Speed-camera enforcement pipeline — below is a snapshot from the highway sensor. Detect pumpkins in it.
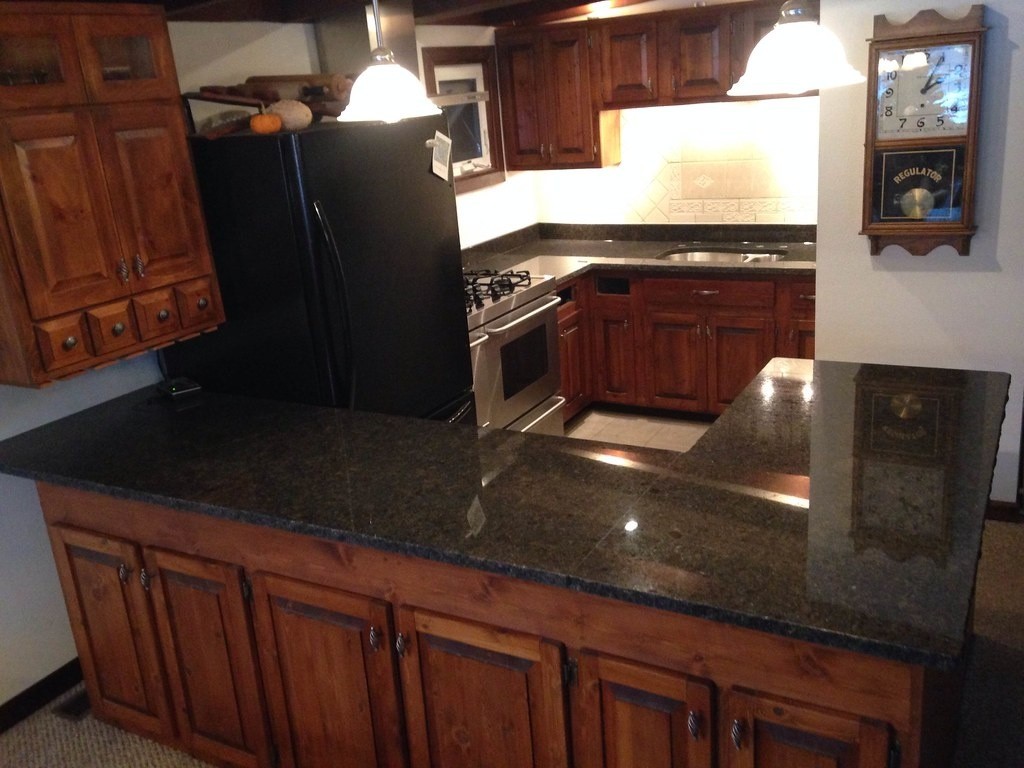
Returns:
[250,101,282,135]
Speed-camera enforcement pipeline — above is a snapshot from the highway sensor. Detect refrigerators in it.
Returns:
[173,113,477,426]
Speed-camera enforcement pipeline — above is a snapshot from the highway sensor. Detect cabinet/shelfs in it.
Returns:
[36,482,934,768]
[494,21,623,170]
[0,0,229,390]
[586,271,816,416]
[555,274,592,424]
[588,0,820,110]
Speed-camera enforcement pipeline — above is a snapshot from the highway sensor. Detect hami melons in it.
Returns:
[262,99,312,131]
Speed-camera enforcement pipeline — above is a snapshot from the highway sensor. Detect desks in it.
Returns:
[0,356,1012,768]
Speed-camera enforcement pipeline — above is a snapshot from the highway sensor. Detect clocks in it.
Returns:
[856,4,994,259]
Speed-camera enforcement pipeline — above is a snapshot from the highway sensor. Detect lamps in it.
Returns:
[335,0,443,124]
[726,0,867,96]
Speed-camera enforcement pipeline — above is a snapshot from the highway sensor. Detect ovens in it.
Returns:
[468,290,565,437]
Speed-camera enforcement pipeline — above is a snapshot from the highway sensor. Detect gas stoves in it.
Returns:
[462,266,557,332]
